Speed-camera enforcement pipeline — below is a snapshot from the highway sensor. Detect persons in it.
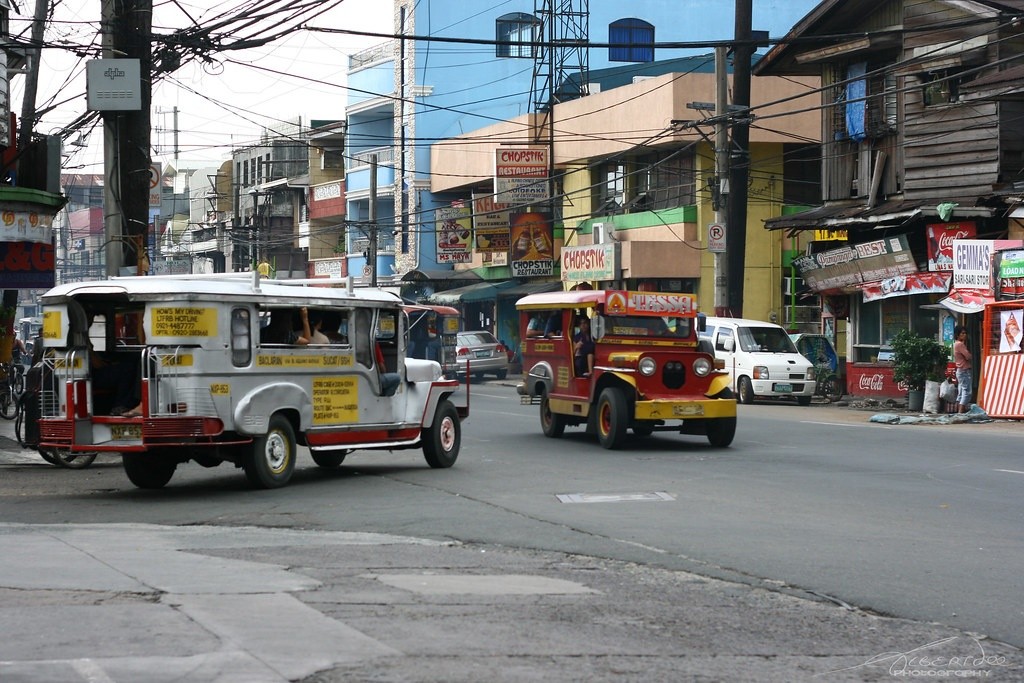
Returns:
[259,306,402,397]
[121,401,143,417]
[11,333,29,382]
[30,328,52,367]
[524,307,668,377]
[953,326,973,415]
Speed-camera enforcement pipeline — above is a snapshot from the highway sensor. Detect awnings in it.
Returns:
[431,279,517,305]
[761,201,949,229]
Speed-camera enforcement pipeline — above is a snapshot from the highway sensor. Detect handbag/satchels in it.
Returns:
[939,375,957,403]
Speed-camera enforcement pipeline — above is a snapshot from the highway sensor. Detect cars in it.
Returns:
[456,330,510,383]
[18,317,42,364]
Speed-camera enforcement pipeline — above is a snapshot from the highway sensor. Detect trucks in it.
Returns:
[33,263,471,491]
[515,289,741,451]
[382,302,460,378]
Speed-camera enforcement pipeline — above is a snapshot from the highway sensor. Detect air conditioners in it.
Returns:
[580,82,601,98]
[592,223,615,245]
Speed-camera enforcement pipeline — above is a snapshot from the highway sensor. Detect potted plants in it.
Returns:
[332,242,345,257]
[888,329,952,409]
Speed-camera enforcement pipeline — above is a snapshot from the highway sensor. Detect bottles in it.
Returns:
[451,221,469,239]
[439,226,447,248]
[516,222,530,257]
[447,224,459,243]
[928,227,940,263]
[532,222,547,252]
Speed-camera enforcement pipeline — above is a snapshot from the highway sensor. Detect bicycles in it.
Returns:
[0,352,28,421]
[801,350,845,402]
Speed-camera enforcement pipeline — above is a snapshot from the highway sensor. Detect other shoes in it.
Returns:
[583,373,591,378]
[122,409,143,417]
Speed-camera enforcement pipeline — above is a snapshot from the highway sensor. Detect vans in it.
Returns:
[692,316,817,407]
